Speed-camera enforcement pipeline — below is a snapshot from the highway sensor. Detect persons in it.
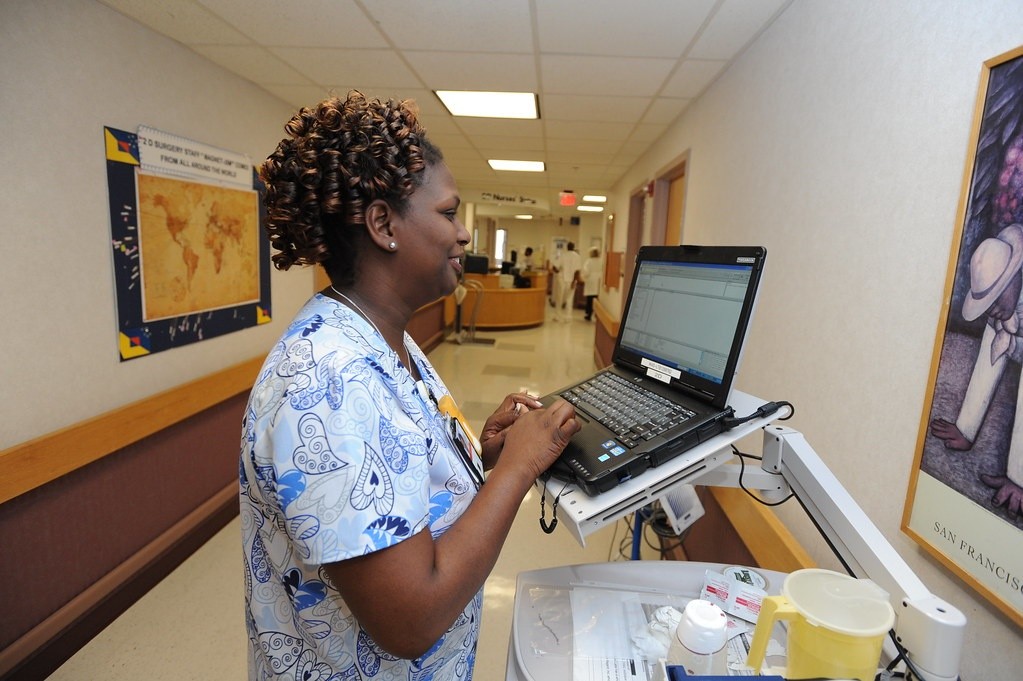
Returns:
[240,88,582,681]
[582,248,603,322]
[458,433,469,456]
[516,247,533,288]
[552,242,582,323]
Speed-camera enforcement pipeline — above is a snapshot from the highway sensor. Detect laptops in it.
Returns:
[521,244,768,498]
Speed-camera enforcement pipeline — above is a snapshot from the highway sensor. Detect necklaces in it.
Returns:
[329,282,412,376]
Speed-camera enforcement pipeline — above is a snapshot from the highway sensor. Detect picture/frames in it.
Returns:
[901,45,1023,627]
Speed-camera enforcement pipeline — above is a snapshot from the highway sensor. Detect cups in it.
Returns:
[666,599,727,675]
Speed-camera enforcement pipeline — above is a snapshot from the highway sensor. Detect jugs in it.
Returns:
[746,568,895,681]
